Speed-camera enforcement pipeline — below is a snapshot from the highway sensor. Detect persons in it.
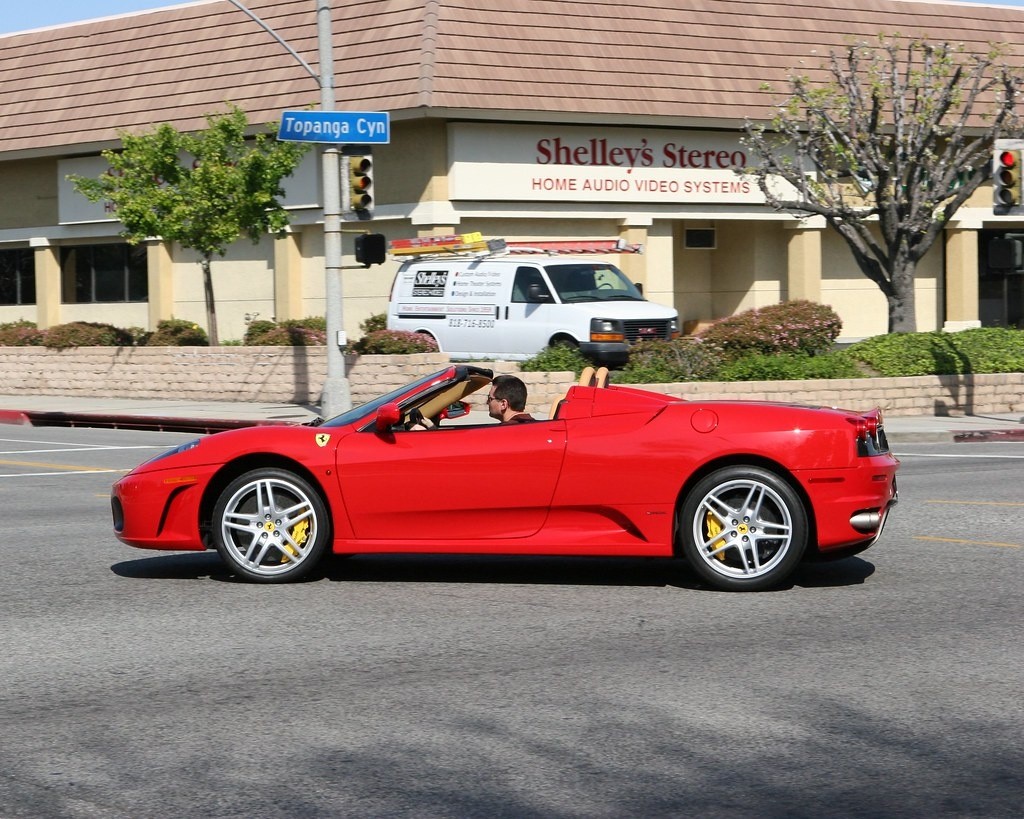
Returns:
[485,374,534,426]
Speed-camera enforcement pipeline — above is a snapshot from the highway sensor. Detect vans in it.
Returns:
[386,249,680,363]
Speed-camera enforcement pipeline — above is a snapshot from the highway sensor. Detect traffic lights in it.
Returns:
[338,150,375,223]
[355,233,385,264]
[993,136,1024,215]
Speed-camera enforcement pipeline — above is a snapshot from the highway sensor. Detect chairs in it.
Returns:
[550,366,610,419]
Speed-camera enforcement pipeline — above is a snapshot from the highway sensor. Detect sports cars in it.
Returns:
[112,363,904,592]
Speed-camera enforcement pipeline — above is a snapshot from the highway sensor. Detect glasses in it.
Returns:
[488,395,510,406]
